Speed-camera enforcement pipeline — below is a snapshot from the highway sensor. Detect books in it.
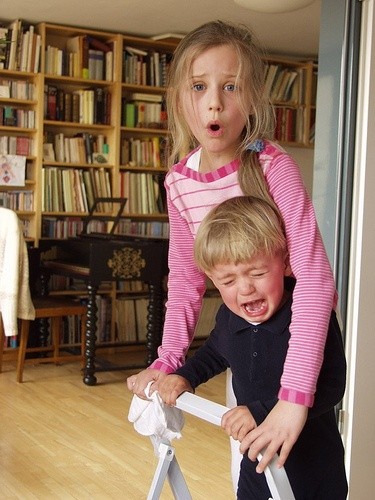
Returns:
[1,165,170,349]
[0,20,318,172]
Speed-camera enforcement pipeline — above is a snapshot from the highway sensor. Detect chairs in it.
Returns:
[0,206,89,384]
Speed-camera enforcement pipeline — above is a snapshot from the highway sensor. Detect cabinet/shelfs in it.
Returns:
[0,21,319,356]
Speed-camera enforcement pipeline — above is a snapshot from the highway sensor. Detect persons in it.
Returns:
[158,196,348,500]
[127,18,342,500]
